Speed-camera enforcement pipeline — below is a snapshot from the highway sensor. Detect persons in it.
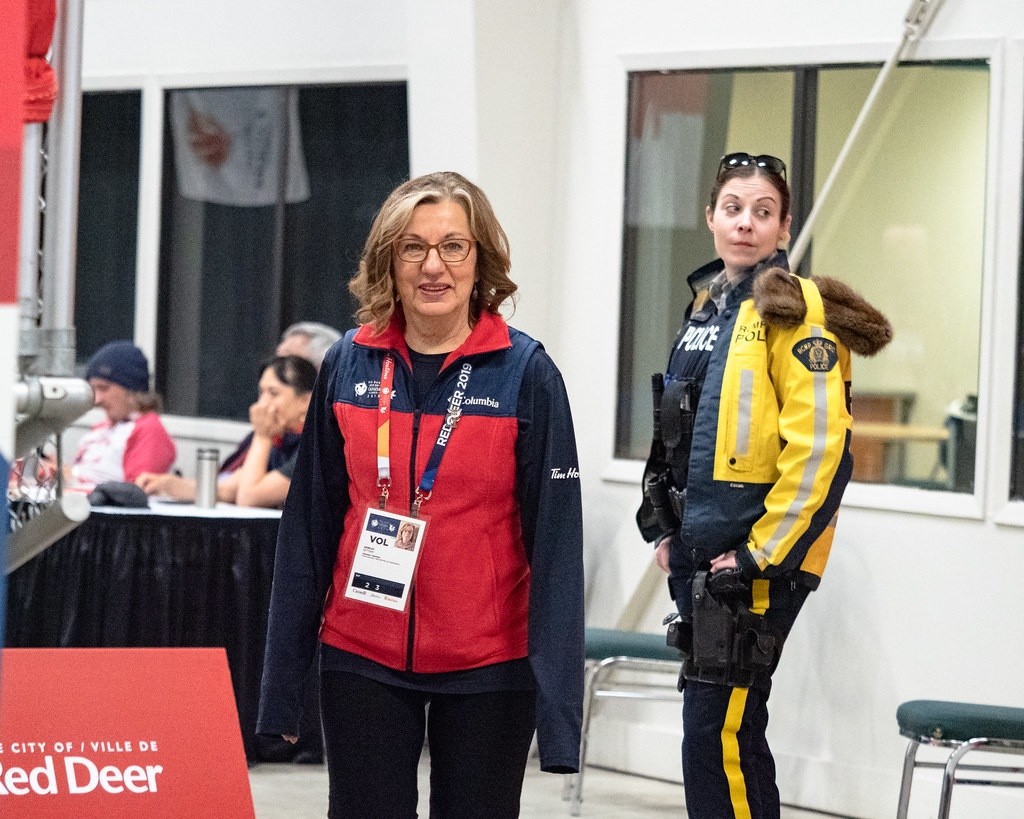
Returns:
[65,341,174,491]
[637,154,856,819]
[134,322,344,507]
[396,523,416,551]
[257,171,586,819]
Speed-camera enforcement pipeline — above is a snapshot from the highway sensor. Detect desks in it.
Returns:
[6,493,322,772]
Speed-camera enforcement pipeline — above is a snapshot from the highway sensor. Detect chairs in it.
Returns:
[895,700,1024,819]
[563,629,681,818]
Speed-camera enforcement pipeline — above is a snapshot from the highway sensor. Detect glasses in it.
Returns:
[716,152,787,184]
[392,238,478,263]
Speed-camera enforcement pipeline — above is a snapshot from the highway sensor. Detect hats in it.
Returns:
[753,268,893,359]
[85,341,150,391]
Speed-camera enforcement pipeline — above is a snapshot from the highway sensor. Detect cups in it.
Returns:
[197,447,219,507]
